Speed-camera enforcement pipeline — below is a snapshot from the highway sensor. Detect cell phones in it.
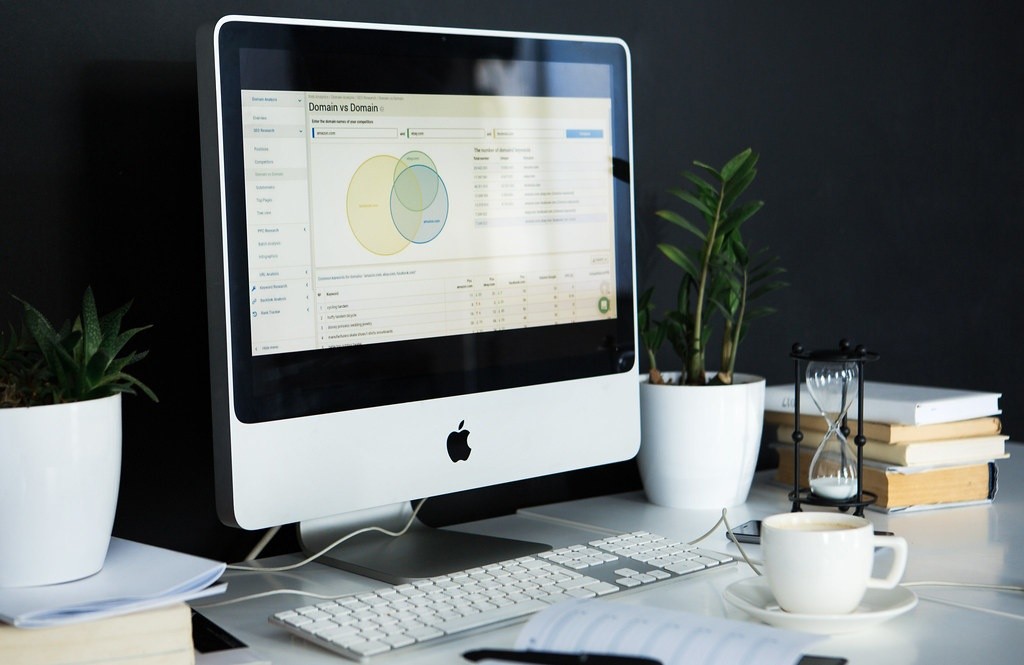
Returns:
[726,520,894,552]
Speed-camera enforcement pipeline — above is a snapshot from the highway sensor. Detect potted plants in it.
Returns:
[0,285,159,590]
[636,146,799,510]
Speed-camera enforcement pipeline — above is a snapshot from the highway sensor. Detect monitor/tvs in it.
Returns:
[195,13,642,588]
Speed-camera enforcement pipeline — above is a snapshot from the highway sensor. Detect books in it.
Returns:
[764,380,1010,513]
[0,580,230,663]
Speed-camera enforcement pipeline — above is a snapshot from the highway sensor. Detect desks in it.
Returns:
[193,437,1024,665]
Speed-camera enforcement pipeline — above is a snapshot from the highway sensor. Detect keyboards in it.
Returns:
[267,531,740,665]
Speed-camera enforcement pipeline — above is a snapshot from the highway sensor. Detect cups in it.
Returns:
[760,511,908,615]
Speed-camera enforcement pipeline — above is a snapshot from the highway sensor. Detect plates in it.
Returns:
[722,575,919,635]
